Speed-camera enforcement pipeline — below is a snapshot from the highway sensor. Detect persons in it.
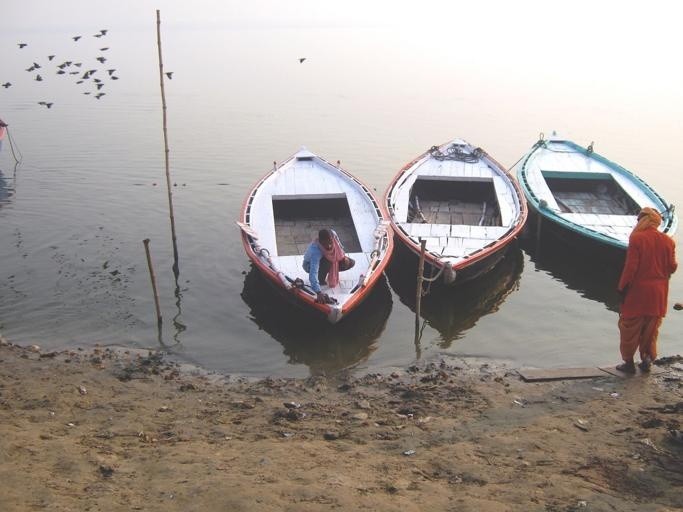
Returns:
[616,208,677,375]
[301,229,355,303]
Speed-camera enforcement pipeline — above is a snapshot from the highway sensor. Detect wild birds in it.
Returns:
[164,71,173,79]
[300,56,306,63]
[24,29,120,108]
[1,81,12,90]
[18,42,27,49]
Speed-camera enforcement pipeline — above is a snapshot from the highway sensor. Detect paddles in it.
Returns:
[236,220,293,290]
[361,221,391,287]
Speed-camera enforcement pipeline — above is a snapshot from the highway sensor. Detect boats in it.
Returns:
[382,136,528,284]
[239,149,395,325]
[518,138,679,275]
